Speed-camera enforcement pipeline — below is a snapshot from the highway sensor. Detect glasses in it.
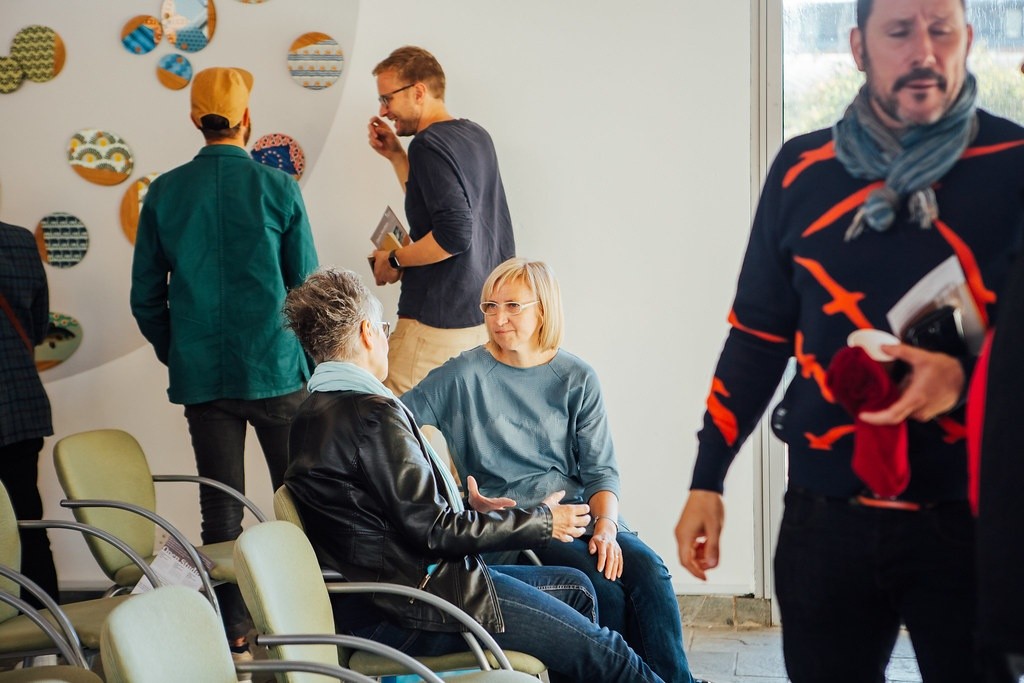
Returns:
[378,84,415,105]
[371,321,390,340]
[479,301,540,315]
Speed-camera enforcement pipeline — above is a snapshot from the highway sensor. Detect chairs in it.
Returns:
[0,422,552,683]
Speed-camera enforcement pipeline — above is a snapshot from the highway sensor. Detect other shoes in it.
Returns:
[230,640,254,662]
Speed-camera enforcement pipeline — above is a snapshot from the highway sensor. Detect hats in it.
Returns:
[191,66,254,131]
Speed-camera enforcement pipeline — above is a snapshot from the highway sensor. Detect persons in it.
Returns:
[283,257,705,683]
[673,0,1024,683]
[0,221,60,671]
[367,46,516,398]
[129,67,320,674]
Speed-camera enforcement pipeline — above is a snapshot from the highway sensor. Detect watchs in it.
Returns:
[388,249,402,270]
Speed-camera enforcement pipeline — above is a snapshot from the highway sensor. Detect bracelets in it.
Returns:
[593,515,619,531]
